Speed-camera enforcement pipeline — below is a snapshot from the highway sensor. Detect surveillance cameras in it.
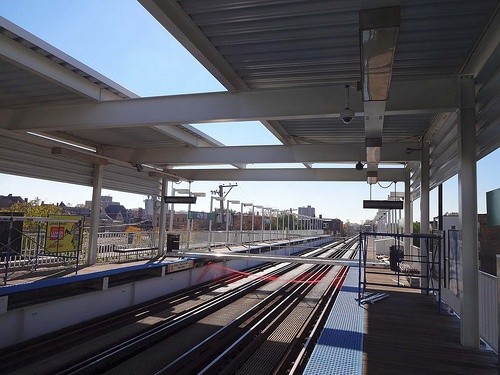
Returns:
[341,86,355,124]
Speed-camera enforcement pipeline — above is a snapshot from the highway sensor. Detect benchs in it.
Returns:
[395,261,421,288]
[113,246,158,263]
